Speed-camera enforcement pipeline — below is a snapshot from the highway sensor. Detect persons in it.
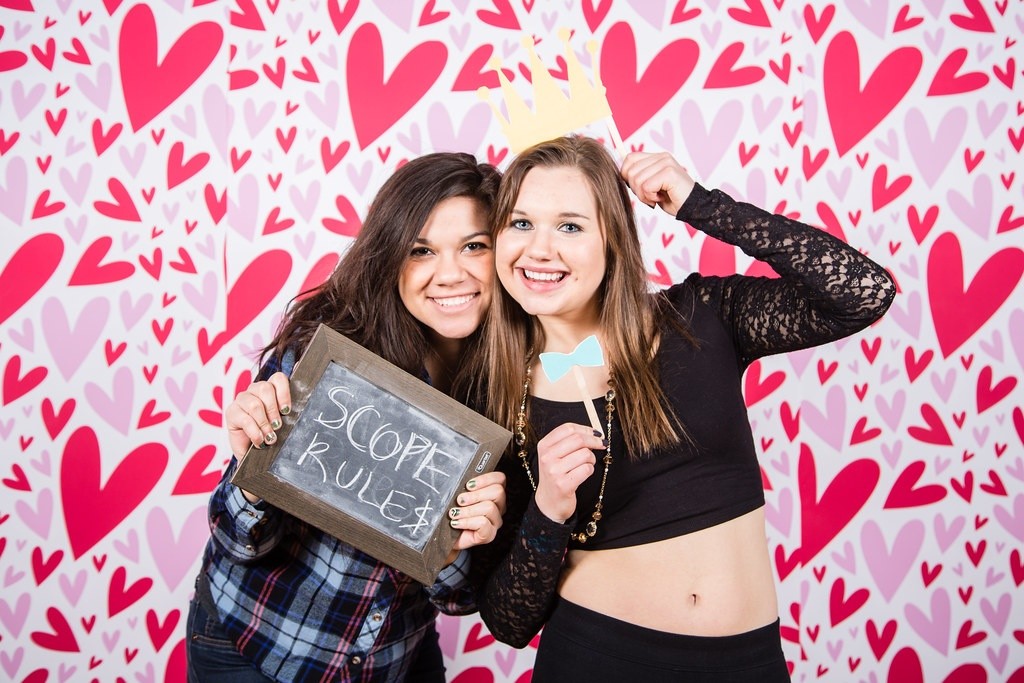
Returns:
[184,150,534,683]
[476,133,897,682]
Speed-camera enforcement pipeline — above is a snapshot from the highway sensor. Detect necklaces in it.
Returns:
[517,343,619,543]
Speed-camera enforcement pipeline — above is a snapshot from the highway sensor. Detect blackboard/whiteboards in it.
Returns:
[226,322,515,592]
